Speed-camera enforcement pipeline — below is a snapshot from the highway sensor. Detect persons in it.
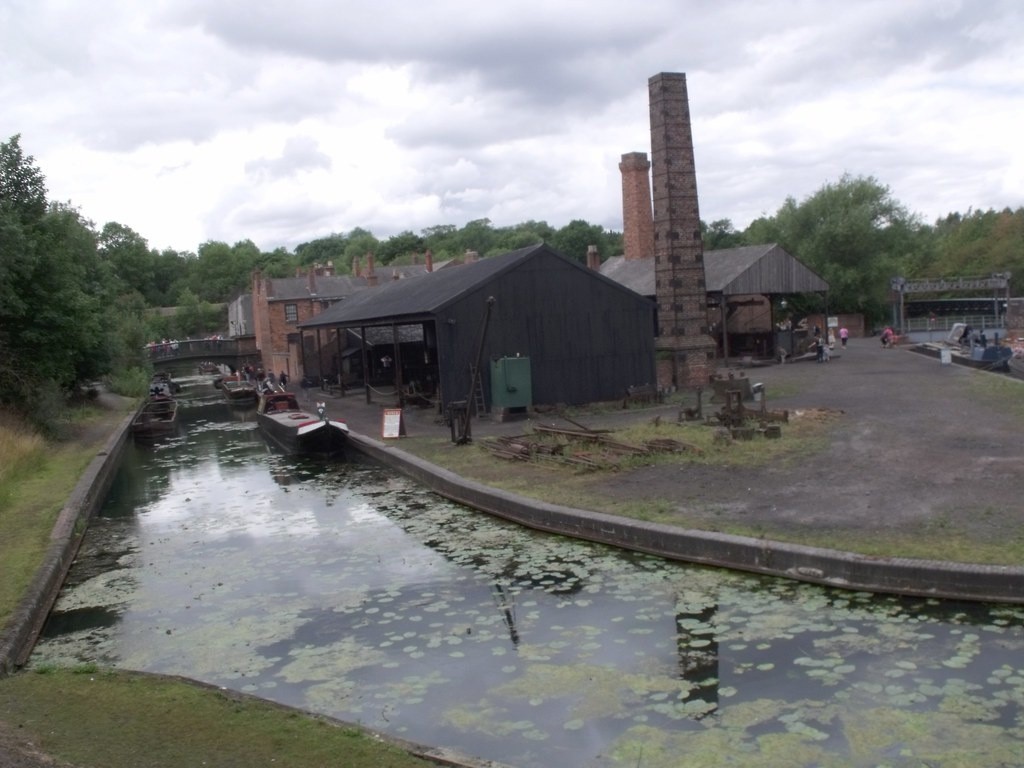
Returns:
[187,335,195,352]
[828,328,836,348]
[815,333,824,363]
[381,356,392,384]
[145,338,182,358]
[879,325,897,348]
[929,311,936,330]
[967,329,987,347]
[204,334,223,350]
[839,325,848,349]
[234,364,290,390]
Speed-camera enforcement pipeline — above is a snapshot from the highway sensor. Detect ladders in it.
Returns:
[469,363,486,417]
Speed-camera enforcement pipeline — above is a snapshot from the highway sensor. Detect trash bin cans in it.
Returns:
[447,400,470,443]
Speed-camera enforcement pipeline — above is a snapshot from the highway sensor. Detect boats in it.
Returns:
[150,370,175,401]
[199,361,222,376]
[132,397,178,435]
[915,322,1014,372]
[222,379,256,400]
[256,391,349,462]
